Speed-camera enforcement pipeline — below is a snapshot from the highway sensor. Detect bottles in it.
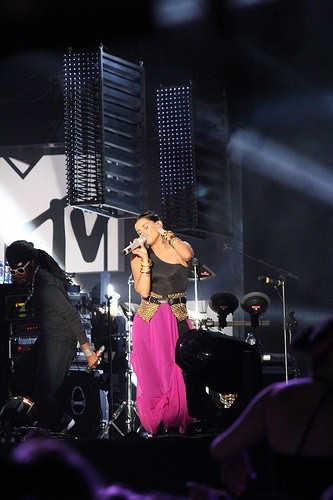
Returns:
[0,261,4,283]
[5,261,12,285]
[100,417,108,437]
[245,333,256,345]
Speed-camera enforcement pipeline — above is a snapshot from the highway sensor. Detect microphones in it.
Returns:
[85,345,105,373]
[122,237,146,255]
[257,276,282,286]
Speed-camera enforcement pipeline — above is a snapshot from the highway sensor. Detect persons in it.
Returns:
[129,211,195,436]
[209,312,333,500]
[4,241,103,432]
[0,437,168,500]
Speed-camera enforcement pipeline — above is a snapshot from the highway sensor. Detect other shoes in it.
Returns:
[55,414,75,438]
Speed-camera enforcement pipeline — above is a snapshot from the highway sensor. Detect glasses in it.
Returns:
[8,261,30,276]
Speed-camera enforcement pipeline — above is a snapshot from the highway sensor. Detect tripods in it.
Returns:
[96,273,141,439]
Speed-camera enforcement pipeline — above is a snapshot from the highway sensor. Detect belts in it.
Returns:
[141,295,187,305]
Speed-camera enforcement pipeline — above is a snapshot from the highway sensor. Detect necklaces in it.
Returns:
[26,265,41,298]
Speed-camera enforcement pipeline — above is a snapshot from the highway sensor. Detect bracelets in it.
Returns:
[83,348,93,357]
[161,230,179,246]
[140,259,152,276]
